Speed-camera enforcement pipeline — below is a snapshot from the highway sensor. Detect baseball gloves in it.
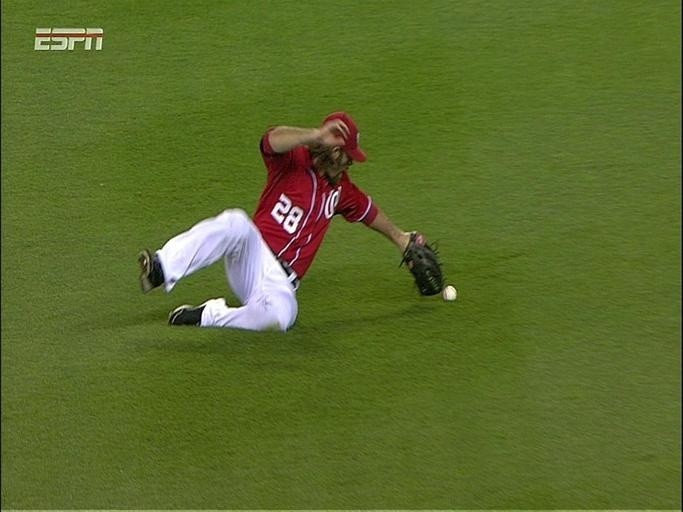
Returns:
[398,231,447,295]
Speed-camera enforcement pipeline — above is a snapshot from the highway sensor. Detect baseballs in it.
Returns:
[443,285,457,302]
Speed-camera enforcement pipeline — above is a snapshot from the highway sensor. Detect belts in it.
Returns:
[279,261,299,288]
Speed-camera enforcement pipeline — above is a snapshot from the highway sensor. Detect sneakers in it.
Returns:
[137,248,164,294]
[168,299,215,328]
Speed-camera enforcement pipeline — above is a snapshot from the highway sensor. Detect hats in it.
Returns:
[323,112,367,162]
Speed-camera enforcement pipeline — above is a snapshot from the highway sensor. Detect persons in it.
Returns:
[136,110,444,334]
